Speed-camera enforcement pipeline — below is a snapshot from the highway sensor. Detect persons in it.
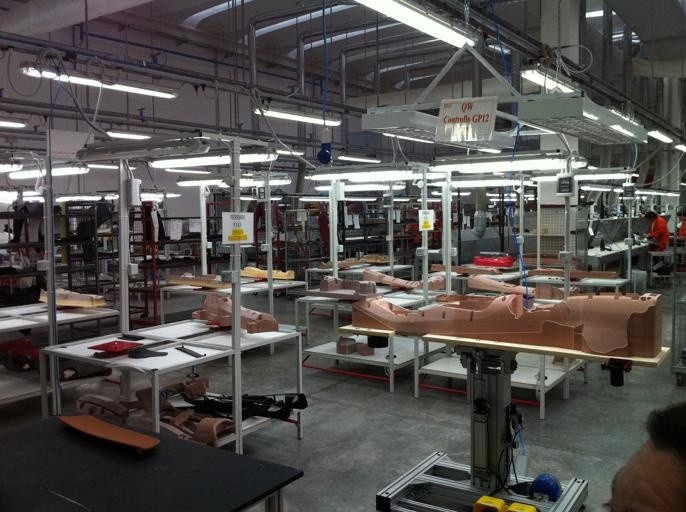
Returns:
[676,211,686,262]
[602,403,686,512]
[646,211,669,280]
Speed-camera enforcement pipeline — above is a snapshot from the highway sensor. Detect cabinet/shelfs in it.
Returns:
[0,304,121,415]
[40,319,302,457]
[296,263,670,418]
[161,271,308,365]
[2,206,148,316]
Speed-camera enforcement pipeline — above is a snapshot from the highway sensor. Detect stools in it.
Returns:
[664,247,684,264]
[648,250,675,289]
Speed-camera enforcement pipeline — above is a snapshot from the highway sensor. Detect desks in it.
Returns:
[589,239,650,273]
[1,415,304,512]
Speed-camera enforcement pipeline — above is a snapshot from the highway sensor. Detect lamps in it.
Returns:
[23,62,180,102]
[355,0,478,50]
[251,104,343,128]
[1,118,682,205]
[585,104,685,153]
[520,65,579,96]
